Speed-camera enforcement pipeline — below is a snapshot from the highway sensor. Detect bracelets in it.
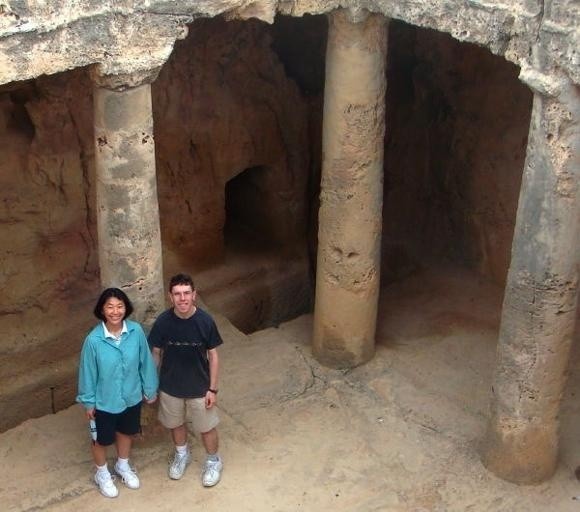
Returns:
[208,387,219,395]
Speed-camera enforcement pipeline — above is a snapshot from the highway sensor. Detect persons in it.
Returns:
[73,286,160,498]
[143,272,225,488]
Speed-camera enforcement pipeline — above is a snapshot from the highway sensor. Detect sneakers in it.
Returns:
[113,461,139,489]
[168,447,192,479]
[201,455,224,487]
[94,470,118,497]
